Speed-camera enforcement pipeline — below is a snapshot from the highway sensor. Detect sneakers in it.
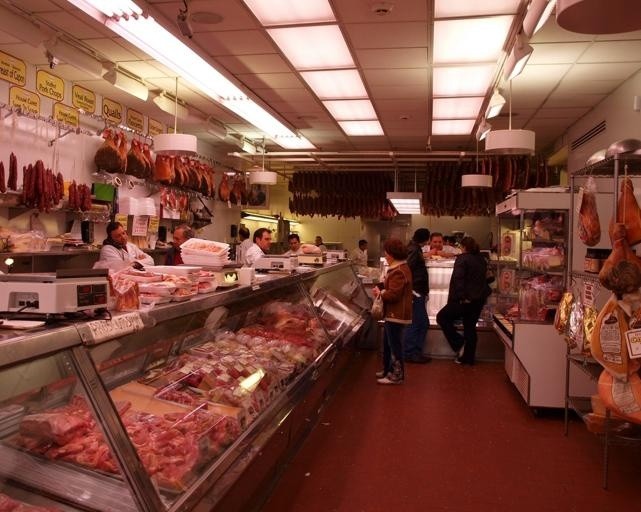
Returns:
[376,371,401,385]
[405,355,431,363]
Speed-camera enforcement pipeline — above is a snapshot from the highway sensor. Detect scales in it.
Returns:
[298,253,327,268]
[255,255,299,274]
[0,269,110,328]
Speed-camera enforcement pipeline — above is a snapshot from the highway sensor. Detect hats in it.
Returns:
[384,238,409,261]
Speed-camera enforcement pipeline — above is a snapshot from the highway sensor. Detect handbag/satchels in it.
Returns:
[370,292,385,320]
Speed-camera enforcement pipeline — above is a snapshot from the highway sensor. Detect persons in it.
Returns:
[282,234,303,255]
[436,236,493,365]
[99,222,155,272]
[349,239,369,267]
[314,235,324,247]
[405,227,433,364]
[421,232,462,258]
[372,238,414,385]
[240,228,272,269]
[238,227,253,262]
[172,225,194,265]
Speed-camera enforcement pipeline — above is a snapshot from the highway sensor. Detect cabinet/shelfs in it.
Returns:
[379,258,504,361]
[493,192,598,417]
[563,155,641,489]
